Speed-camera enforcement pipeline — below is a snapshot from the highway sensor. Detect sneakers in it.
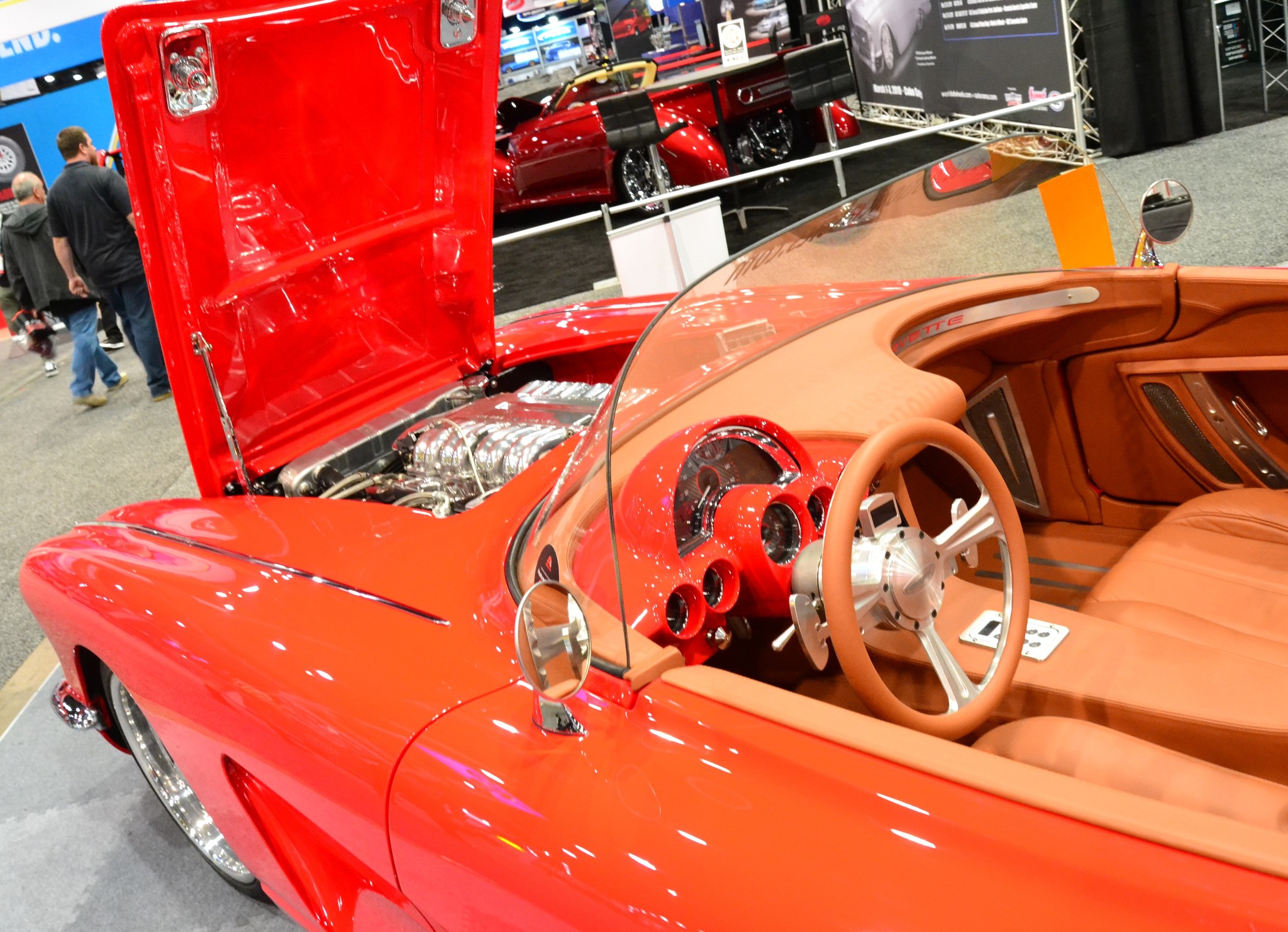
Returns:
[99,338,126,350]
[71,393,108,408]
[106,371,127,391]
[43,359,59,378]
[152,389,172,402]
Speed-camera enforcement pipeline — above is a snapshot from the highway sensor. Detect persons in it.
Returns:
[0,272,60,378]
[90,301,125,352]
[45,128,172,404]
[1,169,130,410]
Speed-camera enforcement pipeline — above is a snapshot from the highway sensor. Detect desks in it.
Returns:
[639,51,791,234]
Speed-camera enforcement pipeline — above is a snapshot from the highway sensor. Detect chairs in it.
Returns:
[971,711,1287,835]
[593,90,690,214]
[1077,488,1288,673]
[782,38,859,201]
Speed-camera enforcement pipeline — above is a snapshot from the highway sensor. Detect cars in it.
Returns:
[18,0,1287,932]
